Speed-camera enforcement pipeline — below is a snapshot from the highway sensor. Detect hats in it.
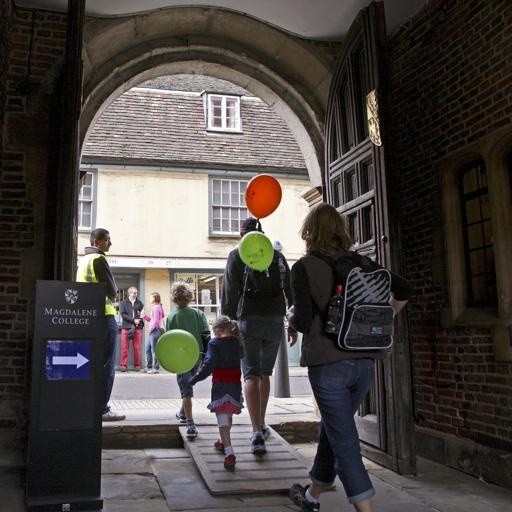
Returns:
[239,216,265,233]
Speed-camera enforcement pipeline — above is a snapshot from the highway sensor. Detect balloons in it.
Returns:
[238,231,275,274]
[244,175,282,220]
[155,329,200,374]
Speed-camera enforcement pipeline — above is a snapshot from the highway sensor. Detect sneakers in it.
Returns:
[289,482,320,511]
[215,439,225,450]
[101,409,126,420]
[175,410,187,420]
[224,454,236,468]
[185,425,198,437]
[120,364,160,374]
[250,424,270,454]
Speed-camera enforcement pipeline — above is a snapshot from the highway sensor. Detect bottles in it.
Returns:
[326,282,343,333]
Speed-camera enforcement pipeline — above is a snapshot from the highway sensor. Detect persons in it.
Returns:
[219,218,298,453]
[284,202,415,511]
[188,316,245,468]
[77,229,125,421]
[165,280,211,439]
[140,291,165,373]
[119,286,145,372]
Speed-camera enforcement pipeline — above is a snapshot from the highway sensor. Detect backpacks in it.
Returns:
[305,249,396,352]
